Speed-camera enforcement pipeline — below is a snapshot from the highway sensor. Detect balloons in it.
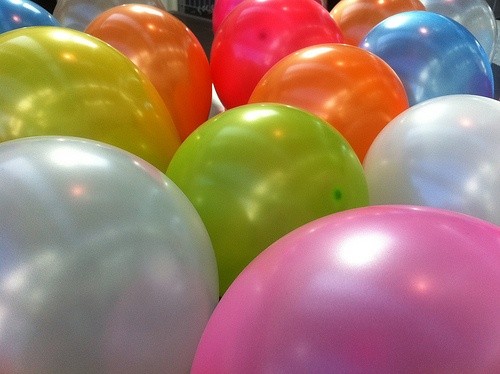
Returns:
[0,135,218,373]
[248,41,410,165]
[212,0,251,41]
[357,10,495,106]
[209,0,343,112]
[86,3,212,145]
[0,25,184,178]
[187,203,500,374]
[361,92,500,231]
[164,100,370,307]
[0,0,66,35]
[328,0,426,46]
[420,0,499,65]
